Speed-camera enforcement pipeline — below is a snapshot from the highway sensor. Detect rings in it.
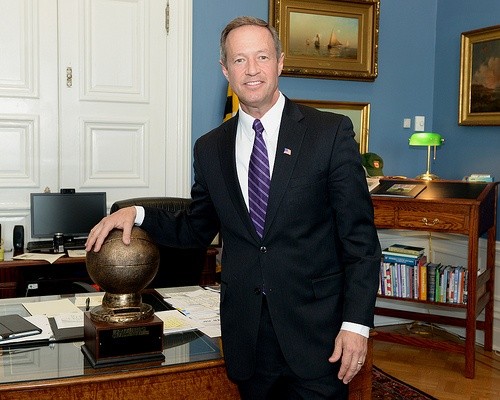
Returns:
[357,361,363,365]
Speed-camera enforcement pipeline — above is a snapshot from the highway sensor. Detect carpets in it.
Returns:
[371,365,441,400]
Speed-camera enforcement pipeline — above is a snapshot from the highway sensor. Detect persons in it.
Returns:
[83,16,382,400]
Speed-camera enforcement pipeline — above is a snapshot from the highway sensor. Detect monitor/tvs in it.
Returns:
[30,192,107,241]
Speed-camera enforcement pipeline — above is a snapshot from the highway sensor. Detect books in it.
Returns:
[366,177,427,199]
[462,173,494,182]
[377,244,482,306]
[0,314,42,341]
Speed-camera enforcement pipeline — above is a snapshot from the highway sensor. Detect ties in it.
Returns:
[247,119,270,239]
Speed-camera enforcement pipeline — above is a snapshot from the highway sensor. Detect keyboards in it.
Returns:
[27,239,87,252]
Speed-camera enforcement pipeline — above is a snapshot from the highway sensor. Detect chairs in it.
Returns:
[111,197,196,288]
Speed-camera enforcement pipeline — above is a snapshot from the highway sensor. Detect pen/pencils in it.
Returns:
[86,297,89,311]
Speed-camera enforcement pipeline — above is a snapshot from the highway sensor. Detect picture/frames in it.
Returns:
[268,0,381,83]
[459,25,500,125]
[290,100,371,157]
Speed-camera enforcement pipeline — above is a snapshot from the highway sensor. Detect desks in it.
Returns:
[0,284,372,400]
[0,250,91,298]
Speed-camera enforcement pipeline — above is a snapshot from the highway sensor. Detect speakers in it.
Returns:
[13,225,24,257]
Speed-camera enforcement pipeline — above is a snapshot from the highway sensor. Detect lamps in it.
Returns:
[408,132,444,181]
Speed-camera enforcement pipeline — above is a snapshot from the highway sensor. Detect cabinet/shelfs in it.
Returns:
[364,177,497,379]
